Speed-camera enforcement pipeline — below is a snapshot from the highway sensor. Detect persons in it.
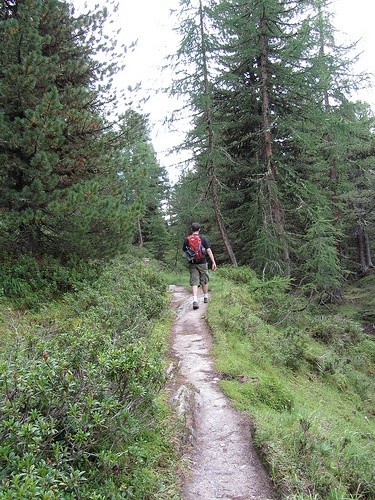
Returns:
[182,223,217,309]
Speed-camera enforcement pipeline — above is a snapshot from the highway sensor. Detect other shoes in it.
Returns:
[204,298,208,302]
[193,302,199,310]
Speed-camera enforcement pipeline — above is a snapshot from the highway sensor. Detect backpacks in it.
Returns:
[187,235,207,264]
